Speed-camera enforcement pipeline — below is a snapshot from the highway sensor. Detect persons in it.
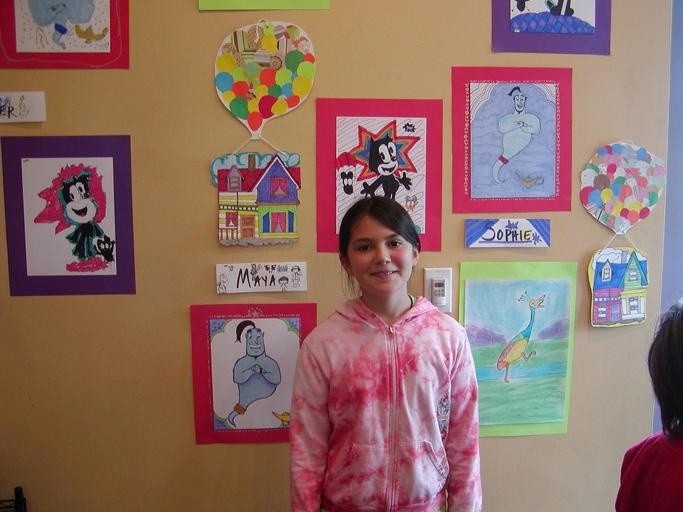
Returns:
[286,194,484,512]
[613,302,683,512]
